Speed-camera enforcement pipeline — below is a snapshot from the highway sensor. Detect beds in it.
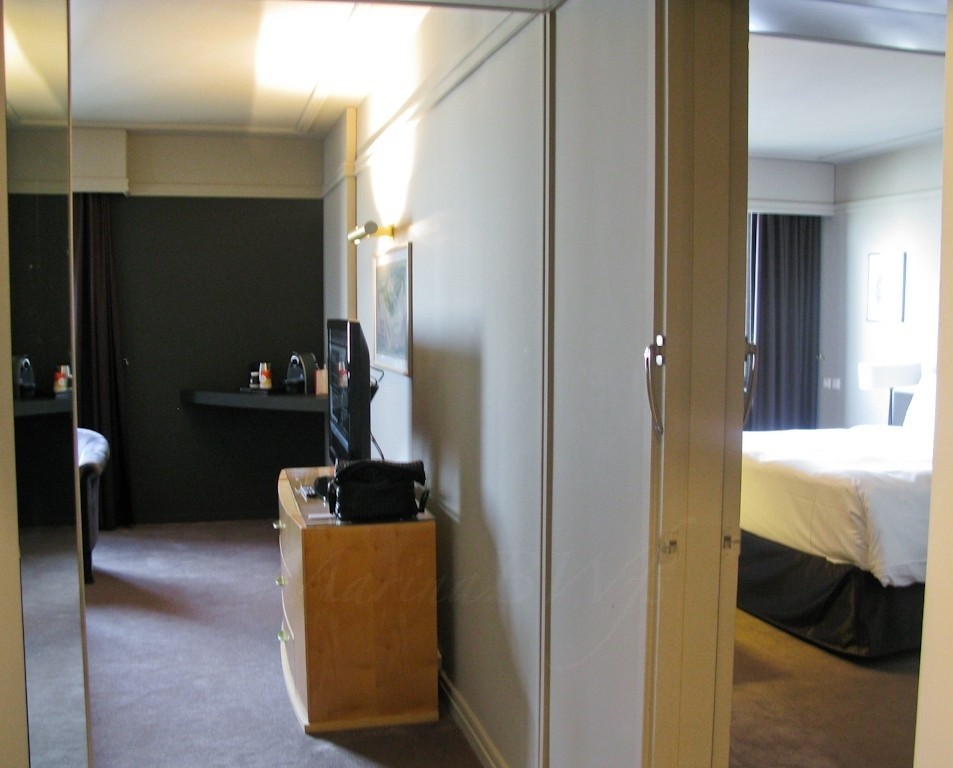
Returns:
[737,360,936,663]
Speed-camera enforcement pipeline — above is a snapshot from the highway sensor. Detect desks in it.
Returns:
[187,388,325,412]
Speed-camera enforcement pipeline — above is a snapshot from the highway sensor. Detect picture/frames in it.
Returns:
[372,242,413,378]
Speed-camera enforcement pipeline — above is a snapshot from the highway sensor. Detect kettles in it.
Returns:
[286,348,320,397]
[12,353,37,402]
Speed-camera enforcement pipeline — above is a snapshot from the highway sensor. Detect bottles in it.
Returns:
[53,372,67,393]
[258,361,273,389]
[250,371,260,389]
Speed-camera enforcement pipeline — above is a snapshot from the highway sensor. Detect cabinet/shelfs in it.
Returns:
[272,466,438,736]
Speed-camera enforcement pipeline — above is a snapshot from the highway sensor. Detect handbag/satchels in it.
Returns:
[327,461,429,523]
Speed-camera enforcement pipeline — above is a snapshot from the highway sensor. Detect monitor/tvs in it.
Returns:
[314,318,371,501]
